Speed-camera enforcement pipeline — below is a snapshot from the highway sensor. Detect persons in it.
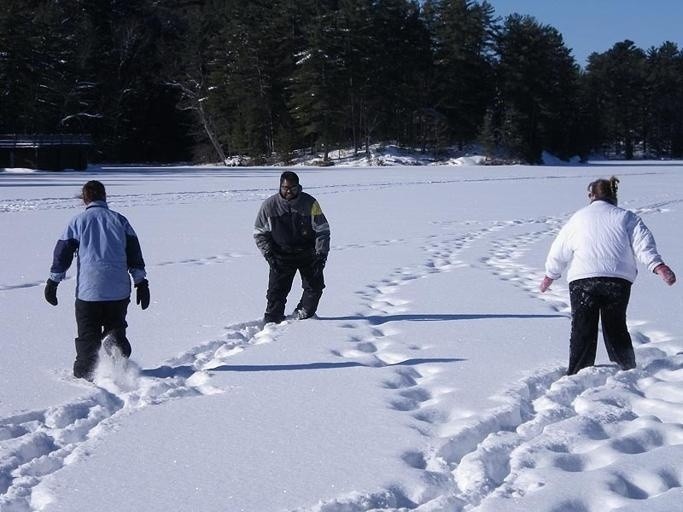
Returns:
[44,180,150,380]
[541,177,676,376]
[254,171,330,323]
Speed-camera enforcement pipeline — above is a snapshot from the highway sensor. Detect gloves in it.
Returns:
[539,275,552,293]
[43,278,59,307]
[134,278,151,311]
[311,255,325,280]
[655,263,677,286]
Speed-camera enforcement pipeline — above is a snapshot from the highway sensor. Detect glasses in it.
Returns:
[281,184,297,191]
